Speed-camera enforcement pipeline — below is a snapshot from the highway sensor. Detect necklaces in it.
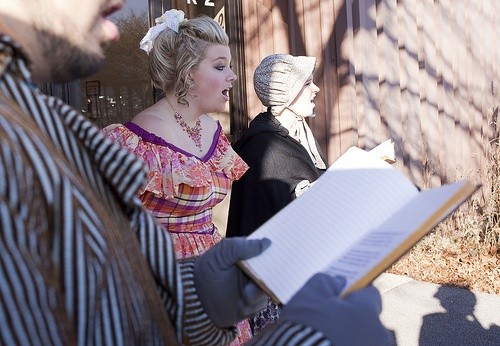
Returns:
[165,94,203,149]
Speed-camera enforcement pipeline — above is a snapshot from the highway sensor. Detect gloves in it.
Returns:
[280,273,394,346]
[193,237,272,328]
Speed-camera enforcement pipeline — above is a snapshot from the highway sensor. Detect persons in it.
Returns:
[0,2,383,346]
[226,53,328,337]
[99,9,253,346]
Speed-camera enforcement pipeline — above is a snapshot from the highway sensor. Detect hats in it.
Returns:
[254,54,317,116]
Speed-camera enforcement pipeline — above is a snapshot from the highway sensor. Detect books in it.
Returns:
[367,139,396,164]
[235,145,482,309]
